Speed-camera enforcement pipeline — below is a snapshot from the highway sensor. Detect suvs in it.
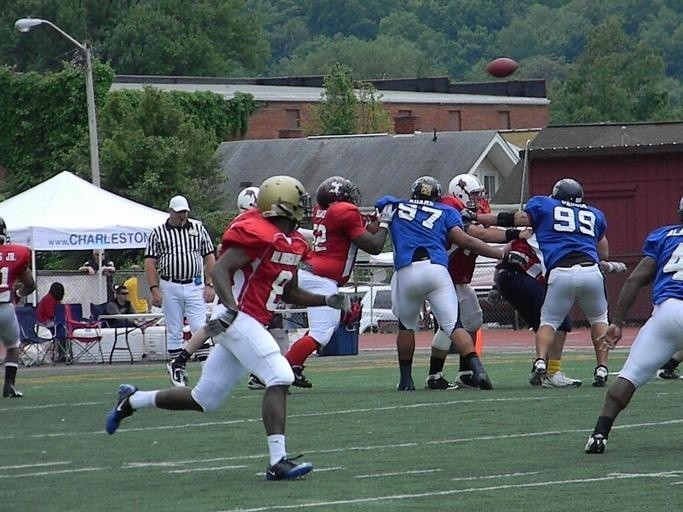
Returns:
[345,286,433,334]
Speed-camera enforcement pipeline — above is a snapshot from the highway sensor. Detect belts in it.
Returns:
[160,275,193,284]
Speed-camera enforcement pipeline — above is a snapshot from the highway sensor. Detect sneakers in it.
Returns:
[454,370,480,390]
[473,371,492,390]
[105,383,137,435]
[592,365,608,387]
[291,365,313,389]
[655,369,683,380]
[528,357,547,387]
[542,370,582,389]
[166,357,191,389]
[265,455,313,481]
[424,372,459,391]
[396,380,416,391]
[584,434,607,454]
[247,373,266,389]
[3,384,24,398]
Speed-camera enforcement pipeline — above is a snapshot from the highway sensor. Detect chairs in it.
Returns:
[56,303,83,362]
[14,306,52,366]
[64,305,104,365]
[90,303,134,327]
[183,318,193,343]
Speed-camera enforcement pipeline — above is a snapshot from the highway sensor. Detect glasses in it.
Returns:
[121,292,129,295]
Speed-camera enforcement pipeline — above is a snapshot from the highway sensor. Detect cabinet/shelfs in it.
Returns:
[274,308,309,328]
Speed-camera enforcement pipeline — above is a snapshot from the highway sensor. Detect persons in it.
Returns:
[461,179,610,388]
[0,218,37,397]
[105,176,352,479]
[377,176,515,390]
[285,176,397,395]
[584,197,682,453]
[143,195,216,370]
[107,287,137,327]
[489,233,627,387]
[656,350,682,380]
[79,249,115,274]
[436,173,533,389]
[167,187,276,389]
[34,282,73,366]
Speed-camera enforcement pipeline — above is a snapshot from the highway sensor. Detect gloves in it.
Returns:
[606,261,628,273]
[375,204,397,229]
[503,251,530,268]
[12,289,22,305]
[487,286,502,305]
[202,306,238,336]
[325,291,352,313]
[460,206,477,224]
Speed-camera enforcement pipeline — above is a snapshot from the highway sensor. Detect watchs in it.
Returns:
[205,282,213,287]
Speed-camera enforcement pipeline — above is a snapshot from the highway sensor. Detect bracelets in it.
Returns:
[149,284,159,289]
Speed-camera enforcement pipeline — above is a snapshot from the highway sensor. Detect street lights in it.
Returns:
[12,19,100,187]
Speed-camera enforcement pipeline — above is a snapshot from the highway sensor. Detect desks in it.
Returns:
[99,314,161,363]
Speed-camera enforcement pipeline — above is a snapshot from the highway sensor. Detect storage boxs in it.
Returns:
[319,321,359,356]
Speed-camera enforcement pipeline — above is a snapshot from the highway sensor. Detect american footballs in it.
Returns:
[486,57,520,79]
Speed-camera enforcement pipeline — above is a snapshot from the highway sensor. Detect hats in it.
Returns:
[169,195,191,213]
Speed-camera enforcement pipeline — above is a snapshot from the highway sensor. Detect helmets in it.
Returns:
[448,173,489,210]
[0,218,6,245]
[677,196,683,221]
[257,175,312,235]
[316,176,362,210]
[237,187,260,213]
[549,178,584,204]
[410,176,444,202]
